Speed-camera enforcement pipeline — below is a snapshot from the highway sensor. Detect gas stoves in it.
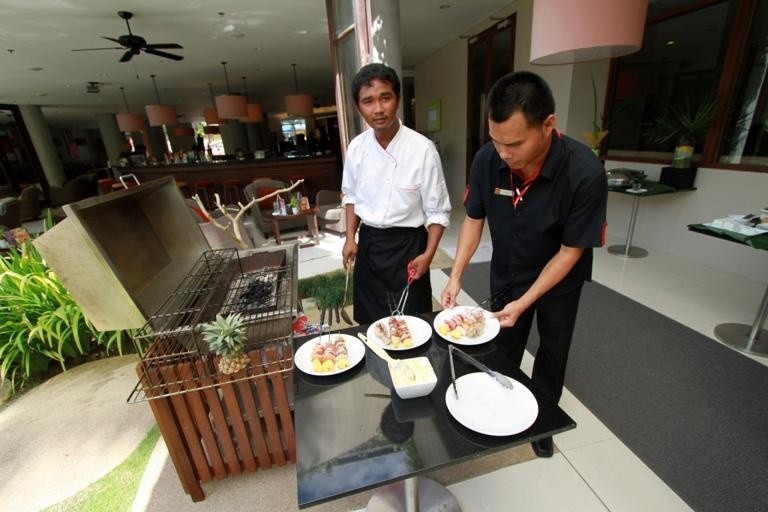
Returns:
[220,265,292,326]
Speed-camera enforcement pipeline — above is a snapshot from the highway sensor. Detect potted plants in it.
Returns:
[640,83,721,169]
[290,195,300,216]
[577,69,638,158]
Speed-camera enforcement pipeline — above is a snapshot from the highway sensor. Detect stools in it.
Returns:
[252,176,272,184]
[101,178,115,194]
[112,182,129,191]
[176,181,191,198]
[97,178,113,195]
[192,178,217,211]
[127,182,137,188]
[218,178,251,218]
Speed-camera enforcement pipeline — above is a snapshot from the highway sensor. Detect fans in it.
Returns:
[71,10,185,63]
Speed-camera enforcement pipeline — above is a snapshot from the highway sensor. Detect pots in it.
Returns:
[606,168,647,188]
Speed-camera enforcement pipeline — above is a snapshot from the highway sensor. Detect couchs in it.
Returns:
[242,179,308,239]
[313,189,347,238]
[48,176,83,206]
[0,196,21,231]
[17,185,42,223]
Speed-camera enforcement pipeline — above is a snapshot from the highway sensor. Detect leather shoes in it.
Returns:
[531,436,553,457]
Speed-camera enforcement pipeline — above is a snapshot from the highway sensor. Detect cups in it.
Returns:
[292,207,300,217]
[631,184,641,191]
[758,210,768,225]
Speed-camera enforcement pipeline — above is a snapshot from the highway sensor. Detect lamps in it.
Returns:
[115,86,142,132]
[144,74,179,127]
[202,83,229,125]
[213,61,249,120]
[528,0,650,65]
[283,63,313,116]
[238,76,264,123]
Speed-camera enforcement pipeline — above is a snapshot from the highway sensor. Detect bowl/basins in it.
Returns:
[386,355,438,399]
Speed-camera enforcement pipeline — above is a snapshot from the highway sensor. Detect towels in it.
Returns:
[713,218,741,233]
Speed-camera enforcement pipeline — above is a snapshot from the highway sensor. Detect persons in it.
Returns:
[441,68,608,457]
[341,63,452,325]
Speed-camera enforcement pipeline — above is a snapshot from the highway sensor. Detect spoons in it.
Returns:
[340,258,353,325]
[356,333,417,383]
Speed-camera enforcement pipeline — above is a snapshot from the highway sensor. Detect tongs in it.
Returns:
[391,260,415,315]
[448,343,514,399]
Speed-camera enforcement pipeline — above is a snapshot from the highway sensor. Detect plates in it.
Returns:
[434,304,501,345]
[727,215,759,224]
[757,224,768,234]
[625,189,647,194]
[366,314,433,351]
[702,220,767,240]
[293,333,366,377]
[444,369,540,437]
